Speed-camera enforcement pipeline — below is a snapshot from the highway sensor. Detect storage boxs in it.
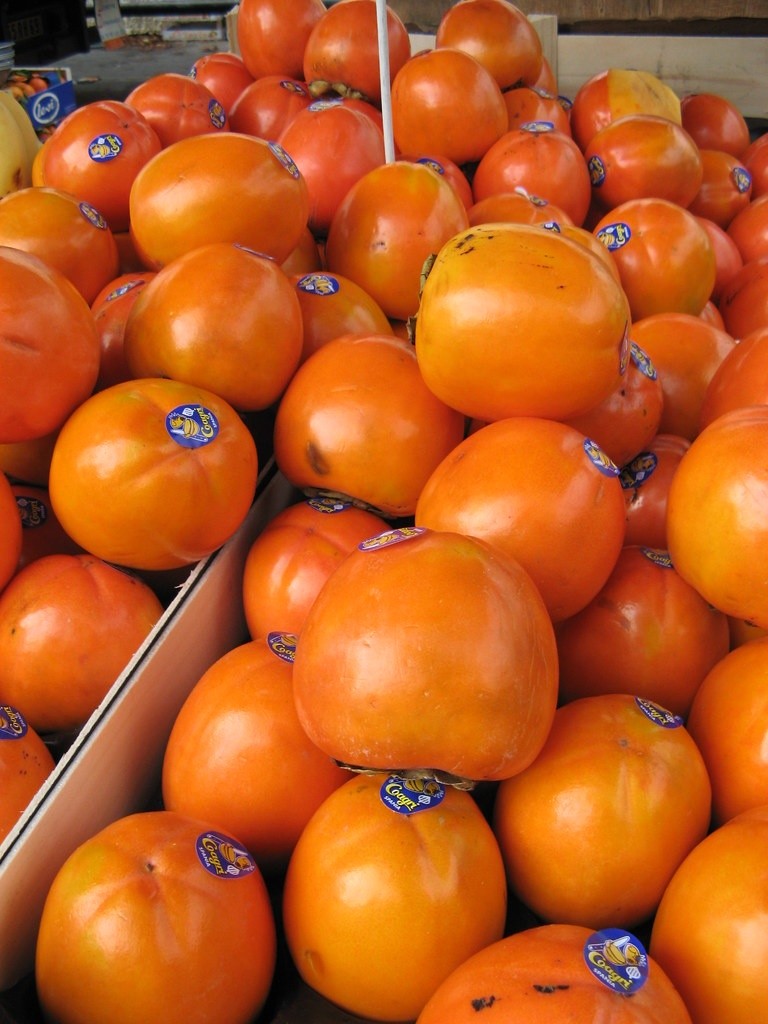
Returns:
[0,15,767,1023]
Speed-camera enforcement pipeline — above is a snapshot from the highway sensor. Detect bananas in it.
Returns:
[0,90,41,200]
